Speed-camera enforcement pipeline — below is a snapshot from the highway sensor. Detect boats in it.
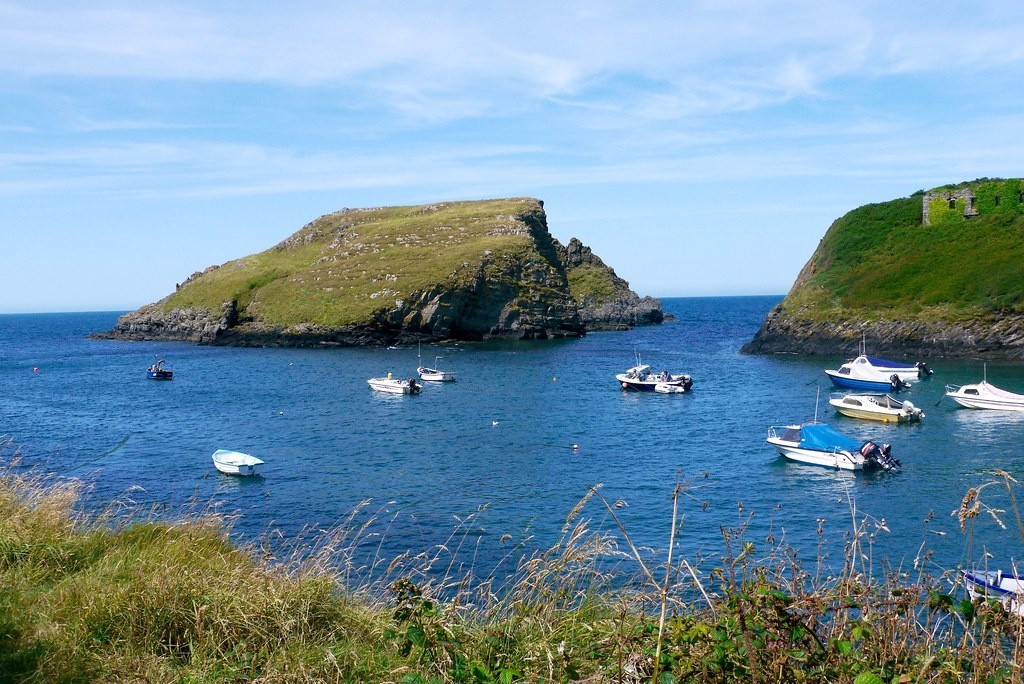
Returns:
[828,388,926,423]
[211,448,265,476]
[825,331,922,391]
[145,355,173,380]
[417,356,458,382]
[654,383,686,394]
[615,342,693,391]
[767,386,902,472]
[366,373,423,395]
[945,362,1024,411]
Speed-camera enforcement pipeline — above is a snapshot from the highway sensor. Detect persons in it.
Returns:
[663,371,673,382]
[632,369,645,381]
